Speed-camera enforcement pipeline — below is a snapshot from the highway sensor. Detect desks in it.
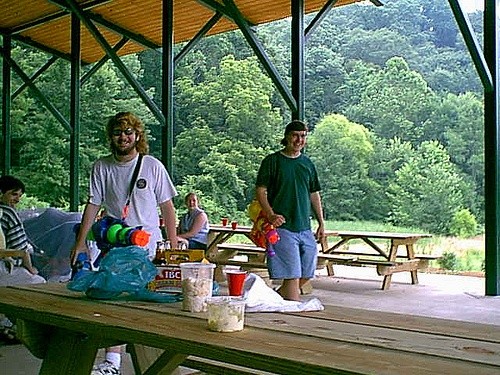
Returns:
[0,279,500,375]
[207,222,274,282]
[307,229,433,291]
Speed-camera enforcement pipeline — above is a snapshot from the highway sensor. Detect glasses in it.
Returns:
[111,128,134,136]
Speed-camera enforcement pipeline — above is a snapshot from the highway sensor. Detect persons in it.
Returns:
[176,193,209,253]
[70,112,178,375]
[256,121,324,301]
[0,176,48,339]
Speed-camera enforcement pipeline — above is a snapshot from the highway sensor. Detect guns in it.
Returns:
[73,215,149,269]
[246,200,280,257]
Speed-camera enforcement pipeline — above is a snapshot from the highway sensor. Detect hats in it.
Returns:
[281,122,306,146]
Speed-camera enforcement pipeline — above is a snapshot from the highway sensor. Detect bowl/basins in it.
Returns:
[205,296,246,332]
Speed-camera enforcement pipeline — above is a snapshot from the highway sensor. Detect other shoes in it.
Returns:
[0,323,20,344]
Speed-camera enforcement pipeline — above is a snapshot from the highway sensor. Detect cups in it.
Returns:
[222,218,228,226]
[225,270,248,296]
[179,262,215,312]
[231,221,238,229]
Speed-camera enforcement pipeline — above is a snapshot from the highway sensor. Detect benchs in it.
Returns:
[217,243,441,274]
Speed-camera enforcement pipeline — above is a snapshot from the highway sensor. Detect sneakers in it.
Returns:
[89,361,120,375]
